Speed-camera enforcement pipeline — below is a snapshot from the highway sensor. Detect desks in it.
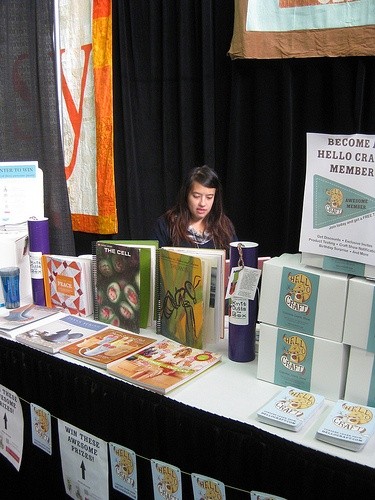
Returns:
[0,308,375,500]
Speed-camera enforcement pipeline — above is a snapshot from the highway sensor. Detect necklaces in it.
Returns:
[190,221,217,249]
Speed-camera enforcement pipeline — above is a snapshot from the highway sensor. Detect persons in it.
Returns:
[132,347,192,381]
[153,165,239,260]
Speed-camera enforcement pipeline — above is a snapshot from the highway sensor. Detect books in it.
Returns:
[315,399,375,452]
[0,239,226,396]
[257,385,325,431]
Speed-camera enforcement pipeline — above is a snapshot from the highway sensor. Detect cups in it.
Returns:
[0,267,20,309]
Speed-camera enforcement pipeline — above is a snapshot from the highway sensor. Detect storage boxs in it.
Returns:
[256,251,375,408]
